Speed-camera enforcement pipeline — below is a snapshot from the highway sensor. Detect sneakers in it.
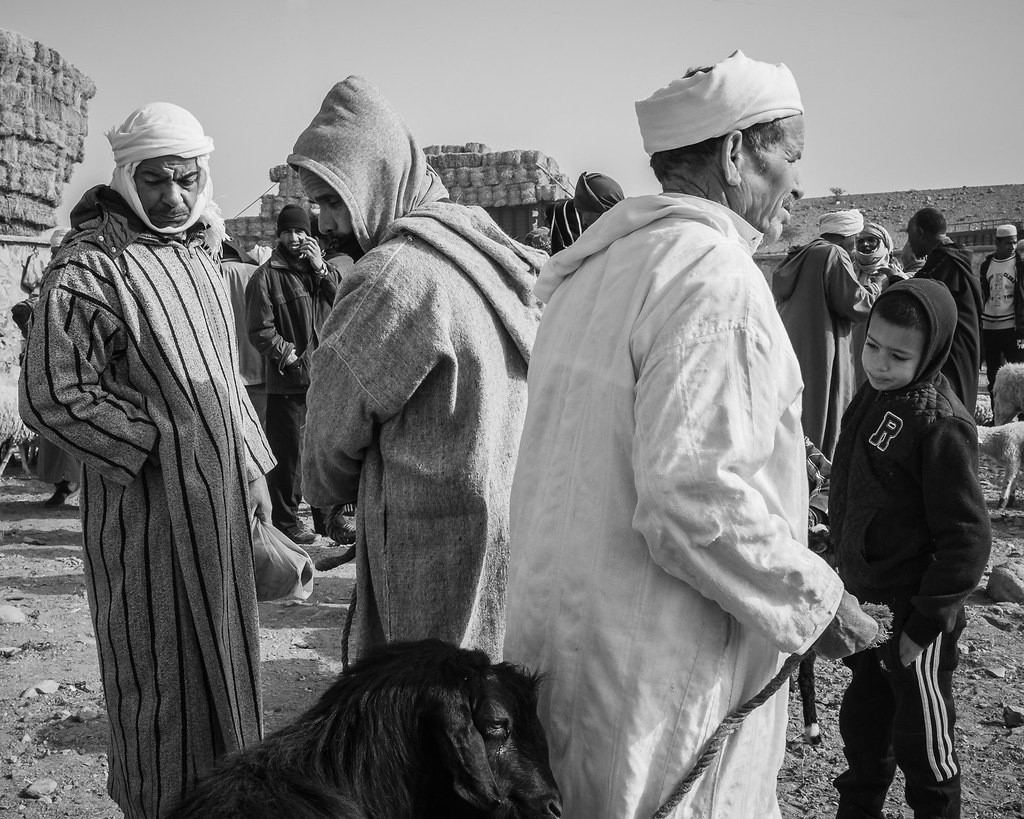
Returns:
[279,522,315,544]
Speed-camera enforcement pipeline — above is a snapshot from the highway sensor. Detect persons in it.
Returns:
[500,52,879,819]
[827,277,993,819]
[293,72,551,662]
[771,207,1024,557]
[524,170,624,256]
[11,204,355,545]
[17,102,280,819]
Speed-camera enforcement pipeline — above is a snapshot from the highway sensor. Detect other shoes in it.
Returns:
[45,487,79,509]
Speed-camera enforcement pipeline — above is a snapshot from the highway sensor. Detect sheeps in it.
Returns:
[977,362,1024,510]
[165,640,563,819]
[0,362,39,475]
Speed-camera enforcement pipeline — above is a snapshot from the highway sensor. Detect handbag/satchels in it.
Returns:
[251,519,313,604]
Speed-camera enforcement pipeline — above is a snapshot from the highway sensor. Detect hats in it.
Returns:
[276,203,311,236]
[997,224,1016,237]
[818,208,864,236]
[50,229,69,247]
